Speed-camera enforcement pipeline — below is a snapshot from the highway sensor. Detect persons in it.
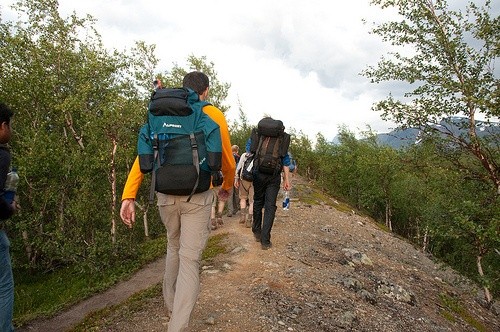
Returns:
[234,153,255,227]
[120,72,236,332]
[210,190,226,231]
[0,100,16,332]
[245,128,290,250]
[227,145,240,217]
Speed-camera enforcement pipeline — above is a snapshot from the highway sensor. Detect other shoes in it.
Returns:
[232,207,239,215]
[239,213,246,224]
[262,241,272,249]
[246,218,252,228]
[216,212,224,225]
[211,218,217,231]
[227,214,232,217]
[256,235,262,242]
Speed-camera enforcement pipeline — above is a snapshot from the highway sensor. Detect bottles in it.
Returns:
[282,187,290,210]
[3,168,19,205]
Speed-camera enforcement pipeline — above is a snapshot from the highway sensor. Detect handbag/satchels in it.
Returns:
[232,153,240,164]
[242,156,255,182]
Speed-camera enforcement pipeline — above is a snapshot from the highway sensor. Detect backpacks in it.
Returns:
[250,117,291,175]
[137,87,224,202]
